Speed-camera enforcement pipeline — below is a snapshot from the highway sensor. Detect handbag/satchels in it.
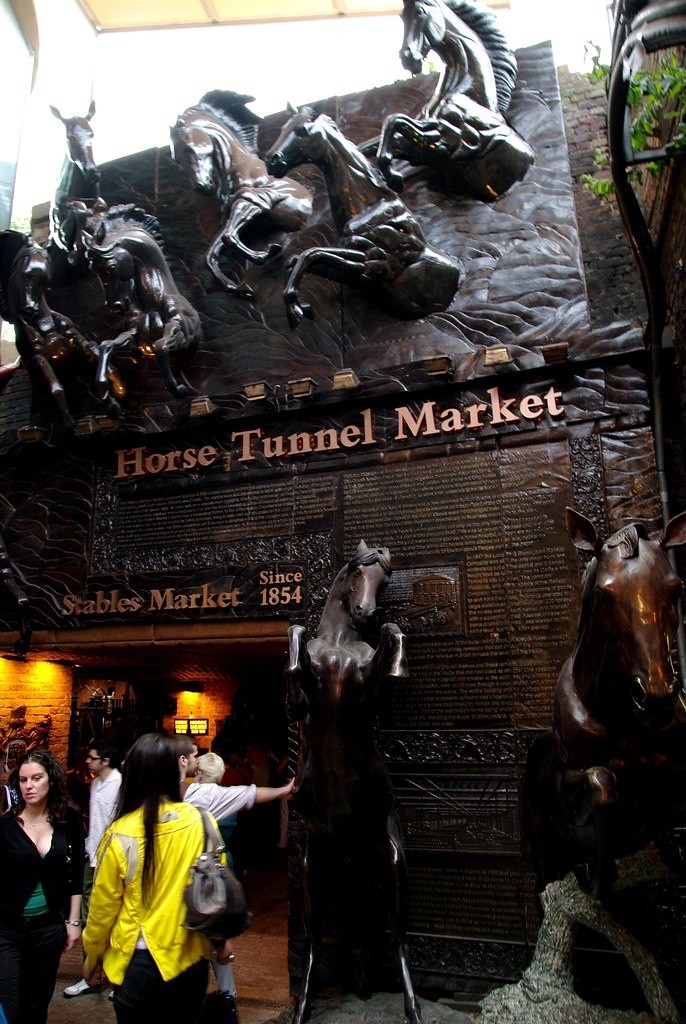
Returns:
[179,805,250,941]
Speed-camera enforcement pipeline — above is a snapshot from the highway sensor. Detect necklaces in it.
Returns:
[24,811,45,826]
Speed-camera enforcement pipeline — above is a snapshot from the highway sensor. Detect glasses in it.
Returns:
[87,753,104,762]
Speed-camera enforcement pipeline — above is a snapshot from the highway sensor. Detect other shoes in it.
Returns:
[107,990,114,998]
[63,978,104,996]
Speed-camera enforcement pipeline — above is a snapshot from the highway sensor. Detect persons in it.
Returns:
[176,735,300,1004]
[0,751,84,1024]
[82,734,234,1024]
[63,739,123,998]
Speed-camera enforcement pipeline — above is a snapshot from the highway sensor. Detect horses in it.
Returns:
[284,535,427,1024]
[0,98,203,436]
[263,100,466,332]
[519,500,686,979]
[169,87,314,300]
[354,0,537,204]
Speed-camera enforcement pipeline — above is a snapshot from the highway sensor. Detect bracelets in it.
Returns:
[65,920,83,925]
